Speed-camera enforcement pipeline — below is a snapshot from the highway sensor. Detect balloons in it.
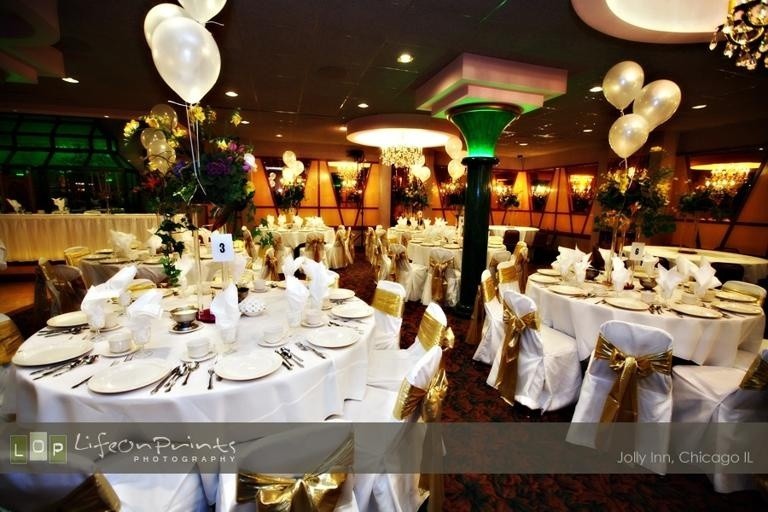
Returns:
[609,113,649,158]
[283,151,305,183]
[412,155,430,183]
[140,105,179,176]
[633,79,682,133]
[179,0,226,22]
[268,172,277,187]
[152,17,221,103]
[603,60,645,110]
[445,136,467,184]
[143,3,189,49]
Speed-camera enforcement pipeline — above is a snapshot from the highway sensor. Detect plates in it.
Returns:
[529,267,762,318]
[82,247,244,265]
[10,279,374,394]
[411,237,503,249]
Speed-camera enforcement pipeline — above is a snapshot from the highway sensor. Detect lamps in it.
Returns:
[345,113,458,148]
